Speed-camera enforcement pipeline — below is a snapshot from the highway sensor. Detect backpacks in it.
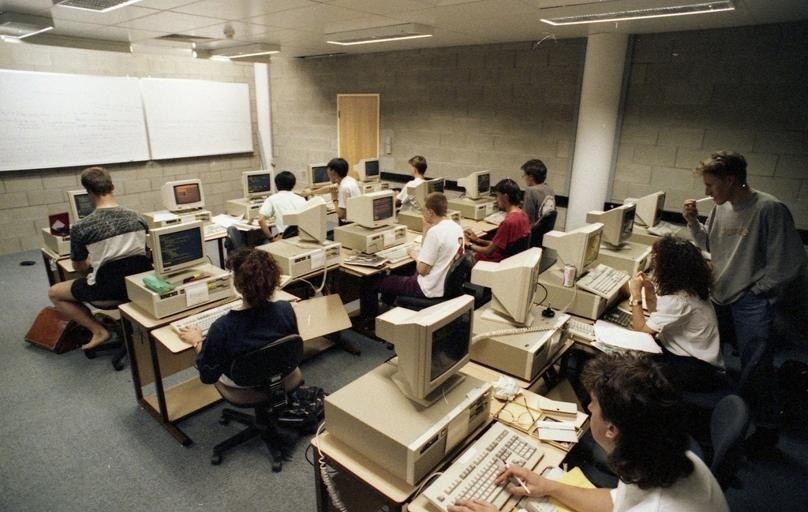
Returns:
[276,383,330,434]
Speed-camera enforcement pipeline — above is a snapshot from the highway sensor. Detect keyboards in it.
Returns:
[576,264,631,300]
[423,424,544,511]
[171,302,240,337]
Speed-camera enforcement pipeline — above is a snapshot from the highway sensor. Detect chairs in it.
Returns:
[681,335,767,421]
[691,394,749,472]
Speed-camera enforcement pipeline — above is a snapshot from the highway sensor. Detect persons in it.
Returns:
[446,350,730,512]
[349,191,465,329]
[251,170,308,247]
[177,249,299,389]
[628,234,725,392]
[463,179,529,284]
[680,150,807,450]
[453,236,465,264]
[393,155,427,211]
[303,157,360,226]
[519,159,556,224]
[48,166,155,351]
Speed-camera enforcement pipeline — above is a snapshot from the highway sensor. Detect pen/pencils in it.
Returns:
[502,460,532,494]
[695,197,715,203]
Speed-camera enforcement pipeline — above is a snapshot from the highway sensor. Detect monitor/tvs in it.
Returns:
[375,295,473,408]
[624,191,666,228]
[586,204,637,246]
[544,223,604,278]
[471,246,543,328]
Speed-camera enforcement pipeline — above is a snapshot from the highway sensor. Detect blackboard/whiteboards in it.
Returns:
[139,77,255,161]
[0,70,151,172]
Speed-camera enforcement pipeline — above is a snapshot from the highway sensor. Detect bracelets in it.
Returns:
[332,199,337,202]
[628,300,642,306]
[193,339,203,349]
[468,242,472,249]
[267,236,273,240]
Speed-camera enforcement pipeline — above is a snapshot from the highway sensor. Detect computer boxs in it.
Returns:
[464,301,571,383]
[597,242,653,277]
[125,263,233,318]
[540,262,619,320]
[324,358,490,485]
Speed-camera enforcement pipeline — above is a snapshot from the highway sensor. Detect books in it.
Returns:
[344,255,389,267]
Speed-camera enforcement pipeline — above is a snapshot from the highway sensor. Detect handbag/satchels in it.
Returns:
[24,305,77,353]
